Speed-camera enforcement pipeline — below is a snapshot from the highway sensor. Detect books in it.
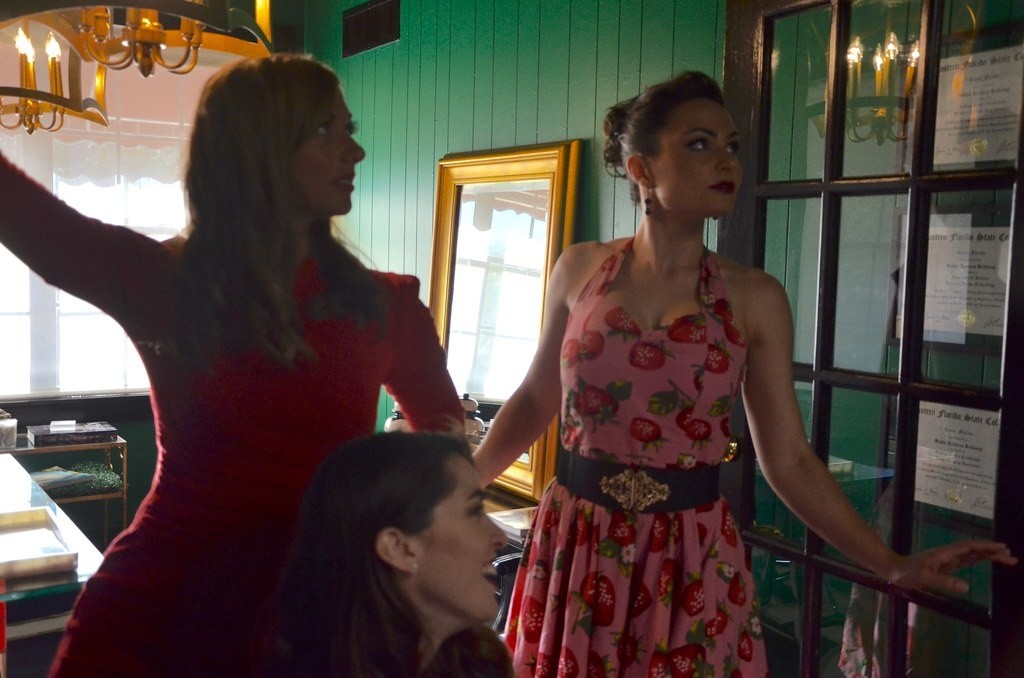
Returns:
[26,419,118,447]
[31,466,95,491]
[487,505,541,543]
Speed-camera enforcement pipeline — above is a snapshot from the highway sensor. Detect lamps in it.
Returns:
[0,0,276,136]
[805,1,977,145]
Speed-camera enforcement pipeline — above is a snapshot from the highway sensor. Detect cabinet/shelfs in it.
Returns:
[0,432,127,678]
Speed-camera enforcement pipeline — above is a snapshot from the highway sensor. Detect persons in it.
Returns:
[840,103,1024,678]
[469,70,1019,678]
[0,54,473,678]
[277,432,524,678]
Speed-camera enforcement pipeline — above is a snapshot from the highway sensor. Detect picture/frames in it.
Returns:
[423,137,586,502]
